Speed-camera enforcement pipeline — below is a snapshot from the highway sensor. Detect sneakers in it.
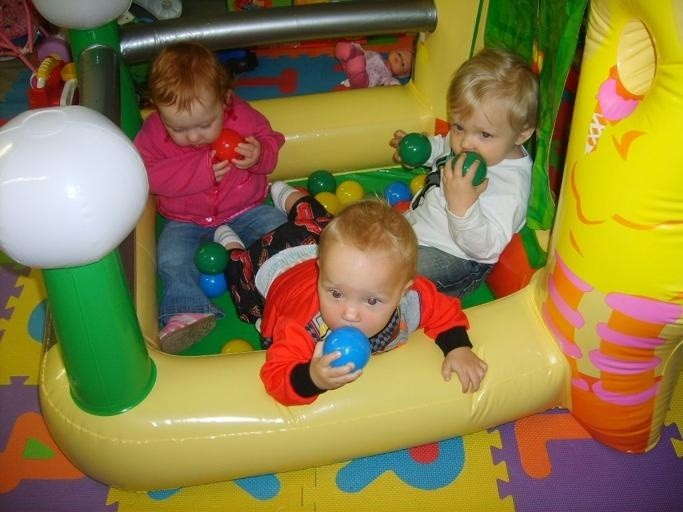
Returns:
[158,313,217,354]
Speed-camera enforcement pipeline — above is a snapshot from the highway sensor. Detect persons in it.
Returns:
[131,41,289,356]
[335,39,415,90]
[212,180,488,407]
[388,46,539,300]
